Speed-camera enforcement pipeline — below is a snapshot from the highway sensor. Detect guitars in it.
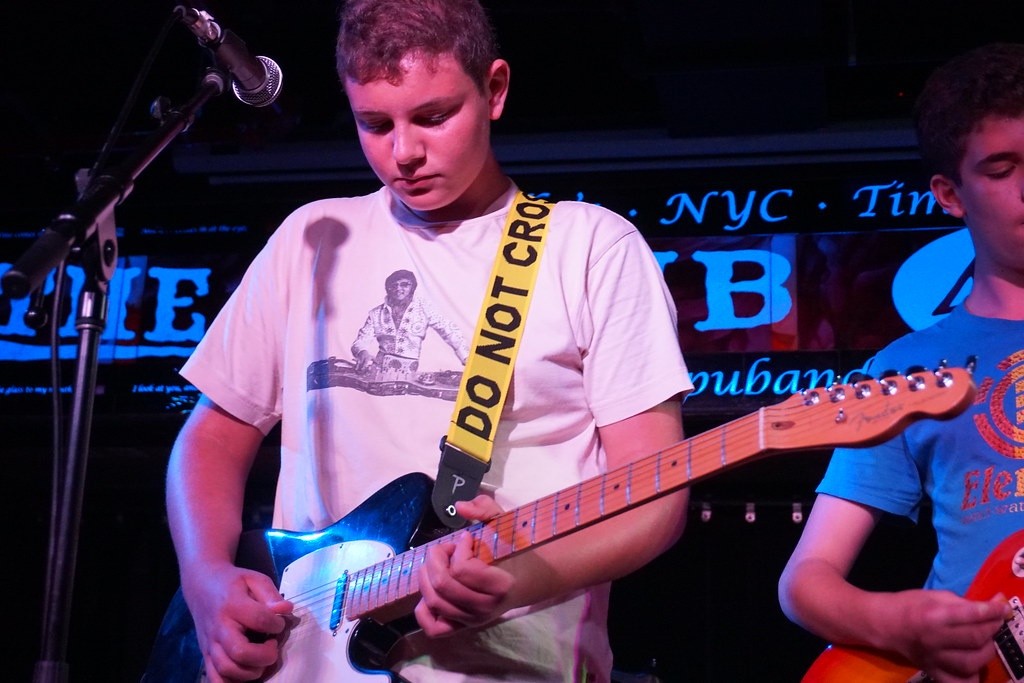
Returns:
[138,352,980,683]
[798,526,1024,683]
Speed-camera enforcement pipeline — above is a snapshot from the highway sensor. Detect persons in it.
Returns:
[778,42,1024,683]
[165,0,692,683]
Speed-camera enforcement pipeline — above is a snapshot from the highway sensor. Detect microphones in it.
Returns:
[172,5,285,108]
